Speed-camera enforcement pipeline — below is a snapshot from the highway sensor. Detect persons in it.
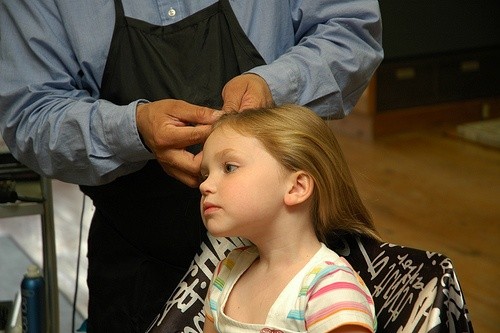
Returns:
[0,0,384,332]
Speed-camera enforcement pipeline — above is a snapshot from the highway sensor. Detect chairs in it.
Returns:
[146,228,474,333]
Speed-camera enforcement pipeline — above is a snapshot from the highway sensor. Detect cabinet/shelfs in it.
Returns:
[367,47,500,141]
